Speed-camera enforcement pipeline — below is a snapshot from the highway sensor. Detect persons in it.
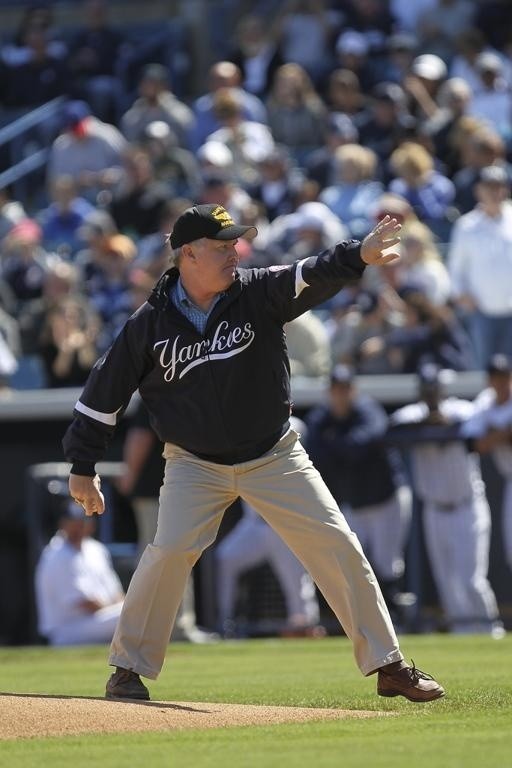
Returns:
[303,365,413,627]
[62,201,446,702]
[36,504,124,645]
[115,391,217,645]
[0,0,512,388]
[459,355,512,567]
[390,363,503,635]
[216,413,323,637]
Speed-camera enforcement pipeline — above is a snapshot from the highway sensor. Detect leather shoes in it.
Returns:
[105,672,150,701]
[377,668,446,702]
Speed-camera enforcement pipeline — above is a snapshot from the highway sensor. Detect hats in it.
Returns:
[170,204,258,249]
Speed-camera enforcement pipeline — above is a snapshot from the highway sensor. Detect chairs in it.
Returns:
[23,465,140,592]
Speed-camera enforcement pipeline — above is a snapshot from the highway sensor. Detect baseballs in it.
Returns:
[82,491,105,512]
